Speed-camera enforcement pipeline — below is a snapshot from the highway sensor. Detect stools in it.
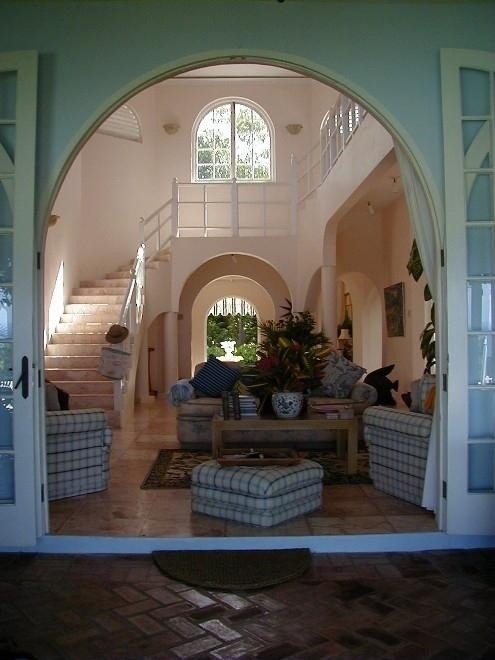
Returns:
[190,459,324,526]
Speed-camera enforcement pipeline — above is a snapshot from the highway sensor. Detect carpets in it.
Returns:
[141,447,373,489]
[153,550,310,591]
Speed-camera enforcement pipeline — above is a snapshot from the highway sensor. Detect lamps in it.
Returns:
[162,124,180,134]
[285,123,303,136]
[392,178,398,193]
[367,201,375,214]
[337,329,351,349]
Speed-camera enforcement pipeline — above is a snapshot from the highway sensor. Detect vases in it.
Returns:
[272,393,304,418]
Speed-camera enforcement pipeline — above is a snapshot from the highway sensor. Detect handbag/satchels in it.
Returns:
[96,347,131,380]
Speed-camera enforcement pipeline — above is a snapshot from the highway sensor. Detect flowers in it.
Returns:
[243,310,332,393]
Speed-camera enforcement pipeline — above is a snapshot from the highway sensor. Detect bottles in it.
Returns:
[221,390,229,421]
[475,334,487,386]
[231,391,241,421]
[481,281,492,331]
[466,281,472,332]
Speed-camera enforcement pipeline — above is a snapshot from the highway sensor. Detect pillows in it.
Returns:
[320,351,366,398]
[189,353,240,397]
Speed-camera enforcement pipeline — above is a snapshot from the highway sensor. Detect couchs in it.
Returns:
[169,362,377,443]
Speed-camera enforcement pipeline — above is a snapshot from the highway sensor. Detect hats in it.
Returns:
[105,324,130,344]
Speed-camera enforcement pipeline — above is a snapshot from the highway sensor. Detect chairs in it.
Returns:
[363,406,433,511]
[45,408,111,501]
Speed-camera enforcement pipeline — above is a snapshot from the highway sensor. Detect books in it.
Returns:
[223,392,261,421]
[306,397,363,421]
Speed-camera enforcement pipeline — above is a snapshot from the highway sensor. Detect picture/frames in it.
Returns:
[384,282,406,337]
